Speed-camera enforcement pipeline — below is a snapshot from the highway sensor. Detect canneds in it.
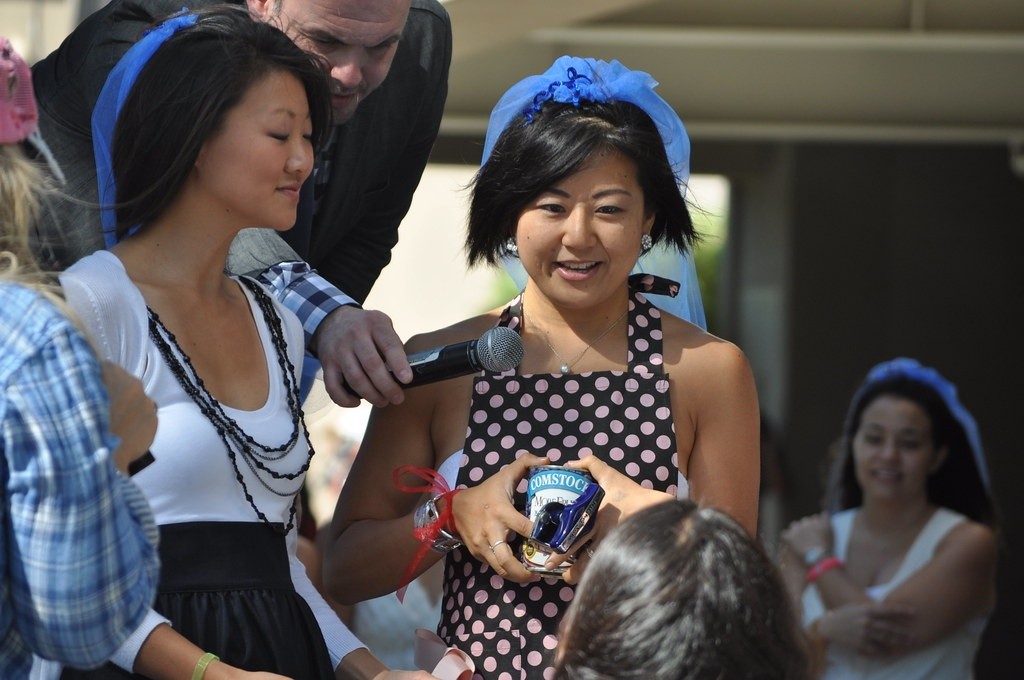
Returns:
[519,465,600,579]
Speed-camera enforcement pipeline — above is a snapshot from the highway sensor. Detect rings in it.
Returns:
[490,540,505,549]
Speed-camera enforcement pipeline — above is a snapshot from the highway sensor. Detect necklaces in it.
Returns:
[521,307,628,376]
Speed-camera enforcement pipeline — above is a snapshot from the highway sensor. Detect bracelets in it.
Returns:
[807,555,843,582]
[392,464,464,605]
[191,652,220,680]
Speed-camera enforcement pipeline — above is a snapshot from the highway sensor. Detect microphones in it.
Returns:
[341,326,524,399]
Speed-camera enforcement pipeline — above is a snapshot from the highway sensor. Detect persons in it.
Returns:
[772,356,1001,680]
[320,55,761,680]
[545,494,826,680]
[0,35,295,680]
[17,0,452,680]
